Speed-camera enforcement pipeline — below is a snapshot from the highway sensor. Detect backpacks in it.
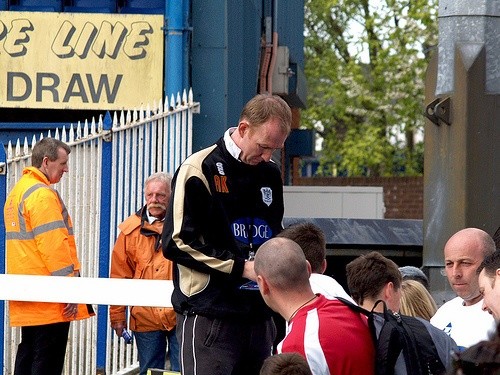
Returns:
[334,297,460,375]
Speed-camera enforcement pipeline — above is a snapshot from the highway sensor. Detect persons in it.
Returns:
[4,138,96,375]
[255,237,375,374]
[110,173,182,375]
[259,352,312,375]
[271,221,500,375]
[162,94,292,375]
